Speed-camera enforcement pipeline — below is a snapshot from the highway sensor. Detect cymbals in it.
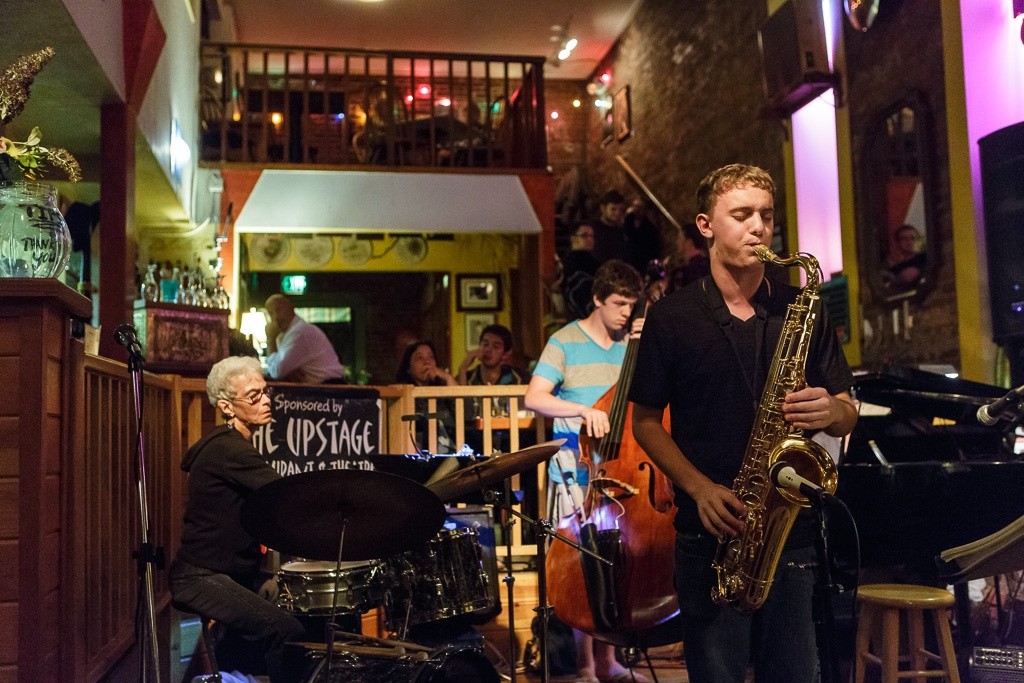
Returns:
[426,437,569,500]
[240,468,448,563]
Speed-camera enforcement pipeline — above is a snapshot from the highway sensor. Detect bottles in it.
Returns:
[134,258,229,309]
[0,179,73,278]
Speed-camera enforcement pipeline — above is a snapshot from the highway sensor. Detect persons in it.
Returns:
[453,324,532,453]
[627,164,858,683]
[674,223,707,285]
[400,342,473,454]
[439,104,487,166]
[560,191,658,319]
[889,225,926,282]
[169,356,310,683]
[524,260,647,683]
[265,294,348,385]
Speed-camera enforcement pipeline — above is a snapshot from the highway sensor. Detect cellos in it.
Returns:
[545,254,684,683]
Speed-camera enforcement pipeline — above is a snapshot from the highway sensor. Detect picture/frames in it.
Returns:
[455,273,503,311]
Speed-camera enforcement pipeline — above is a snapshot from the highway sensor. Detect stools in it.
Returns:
[855,584,960,683]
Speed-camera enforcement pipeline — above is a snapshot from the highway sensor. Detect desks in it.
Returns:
[377,112,479,165]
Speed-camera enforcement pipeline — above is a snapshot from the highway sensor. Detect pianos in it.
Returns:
[830,360,1024,683]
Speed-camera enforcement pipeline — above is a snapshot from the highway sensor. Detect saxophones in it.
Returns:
[709,244,839,615]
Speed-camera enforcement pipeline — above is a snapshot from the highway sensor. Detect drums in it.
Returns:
[308,636,504,683]
[378,526,501,636]
[276,557,390,619]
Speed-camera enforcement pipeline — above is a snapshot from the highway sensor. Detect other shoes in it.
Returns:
[597,668,650,683]
[573,672,599,683]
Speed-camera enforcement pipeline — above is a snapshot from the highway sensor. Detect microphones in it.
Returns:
[976,385,1024,426]
[400,412,449,421]
[768,460,824,497]
[113,322,142,361]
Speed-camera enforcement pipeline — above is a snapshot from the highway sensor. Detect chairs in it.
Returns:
[199,85,255,160]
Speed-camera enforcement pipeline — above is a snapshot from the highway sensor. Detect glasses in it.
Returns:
[221,385,273,405]
